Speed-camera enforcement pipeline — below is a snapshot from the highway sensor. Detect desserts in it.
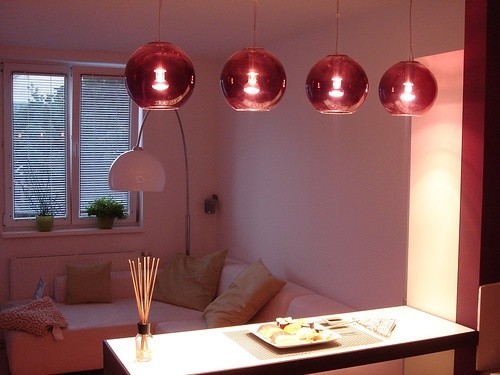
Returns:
[258,316,330,345]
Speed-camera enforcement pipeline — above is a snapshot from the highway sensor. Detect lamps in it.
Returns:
[220,0,287,111]
[305,0,369,116]
[205,194,218,214]
[379,0,438,117]
[109,110,190,256]
[125,0,196,110]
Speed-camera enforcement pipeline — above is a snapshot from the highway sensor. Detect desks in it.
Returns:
[104,306,480,375]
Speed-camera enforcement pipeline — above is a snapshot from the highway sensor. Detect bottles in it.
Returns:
[135,321,153,362]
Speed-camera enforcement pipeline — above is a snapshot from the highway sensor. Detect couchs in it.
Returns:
[5,258,350,375]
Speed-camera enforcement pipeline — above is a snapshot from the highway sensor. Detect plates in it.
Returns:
[249,320,342,349]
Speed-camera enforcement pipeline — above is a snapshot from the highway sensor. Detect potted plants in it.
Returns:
[87,196,128,229]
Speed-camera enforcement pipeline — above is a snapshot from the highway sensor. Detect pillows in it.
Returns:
[153,248,228,311]
[202,258,287,329]
[63,261,114,304]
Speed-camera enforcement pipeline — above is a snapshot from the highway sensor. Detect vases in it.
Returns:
[35,214,54,232]
